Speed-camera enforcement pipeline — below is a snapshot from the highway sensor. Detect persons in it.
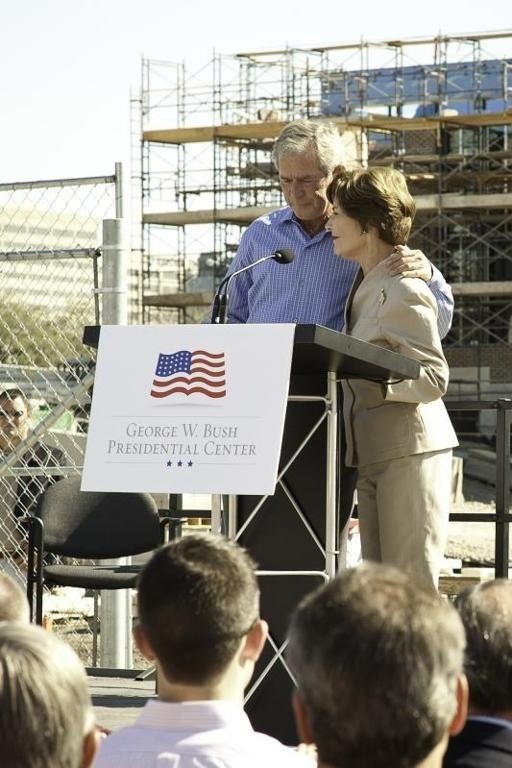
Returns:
[82,533,317,768]
[1,622,99,767]
[281,561,470,768]
[325,163,459,588]
[1,574,30,626]
[1,389,67,592]
[201,119,455,746]
[442,578,512,768]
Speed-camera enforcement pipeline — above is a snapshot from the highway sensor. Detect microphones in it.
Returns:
[209,250,295,323]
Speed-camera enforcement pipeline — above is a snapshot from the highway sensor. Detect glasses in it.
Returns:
[0,410,23,417]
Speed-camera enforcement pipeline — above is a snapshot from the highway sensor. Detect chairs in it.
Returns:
[26,476,188,694]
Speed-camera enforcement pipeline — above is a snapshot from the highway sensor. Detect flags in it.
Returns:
[150,350,226,401]
